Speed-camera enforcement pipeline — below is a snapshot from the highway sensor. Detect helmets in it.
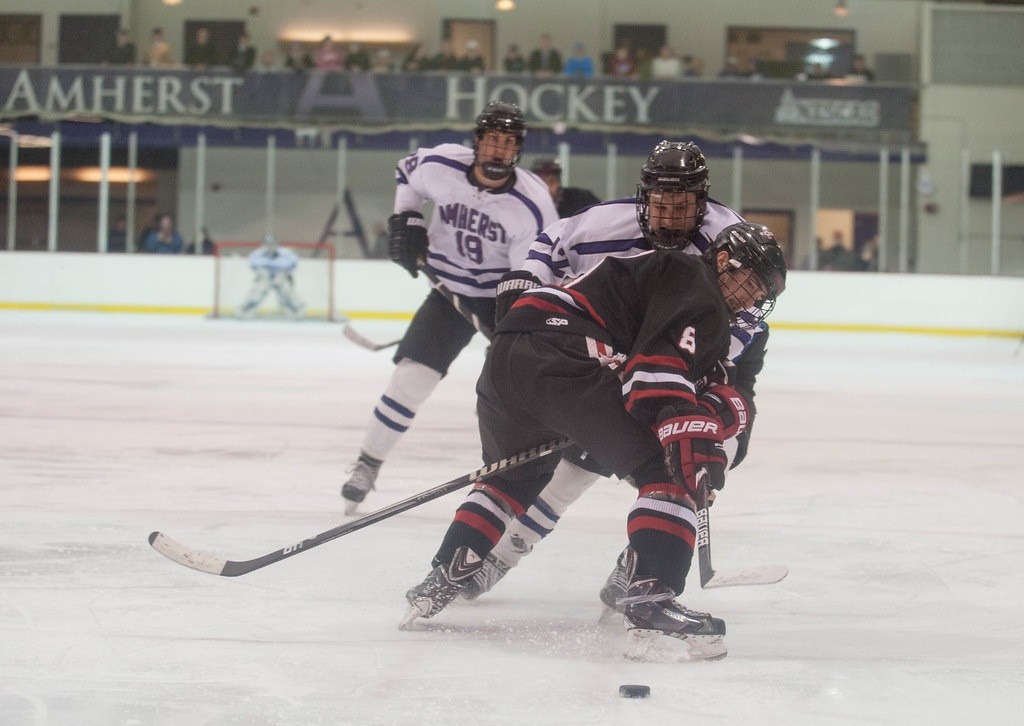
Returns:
[475,101,525,145]
[636,139,711,200]
[531,157,563,174]
[715,222,787,301]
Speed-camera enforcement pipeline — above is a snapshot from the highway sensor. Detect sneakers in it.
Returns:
[600,545,726,636]
[462,553,510,600]
[341,449,383,504]
[405,546,483,618]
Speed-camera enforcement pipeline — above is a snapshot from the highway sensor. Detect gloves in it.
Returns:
[655,403,727,506]
[385,210,429,278]
[698,384,749,439]
[495,270,542,325]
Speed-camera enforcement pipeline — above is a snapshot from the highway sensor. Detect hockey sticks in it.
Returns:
[148,431,572,577]
[340,323,407,352]
[414,259,500,343]
[693,465,791,590]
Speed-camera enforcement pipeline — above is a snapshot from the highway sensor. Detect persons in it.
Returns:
[338,98,561,504]
[403,222,787,638]
[113,29,872,78]
[458,140,769,617]
[108,215,214,254]
[806,227,879,270]
[528,156,601,217]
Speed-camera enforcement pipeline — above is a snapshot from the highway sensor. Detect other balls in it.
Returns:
[618,685,651,700]
